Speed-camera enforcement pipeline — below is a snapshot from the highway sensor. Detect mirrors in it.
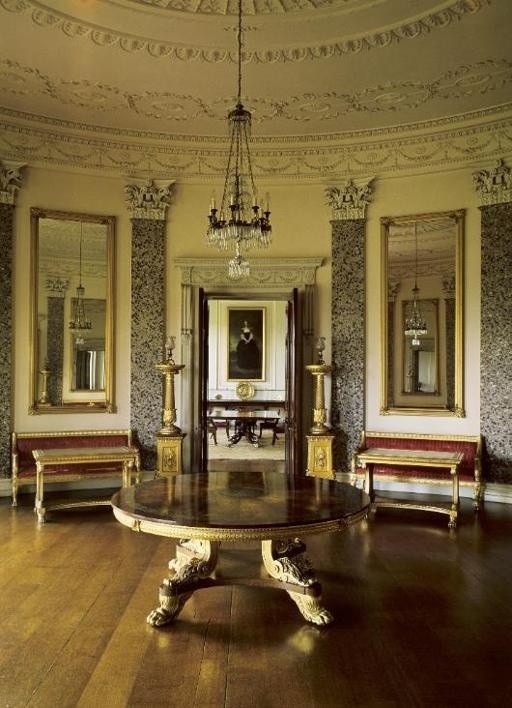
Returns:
[378,208,466,418]
[30,206,116,416]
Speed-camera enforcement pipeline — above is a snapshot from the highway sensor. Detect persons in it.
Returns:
[236,328,259,370]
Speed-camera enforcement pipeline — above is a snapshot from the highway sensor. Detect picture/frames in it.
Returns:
[227,306,266,381]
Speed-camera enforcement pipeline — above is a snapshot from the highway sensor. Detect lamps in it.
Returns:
[205,1,274,278]
[404,222,427,346]
[66,221,93,345]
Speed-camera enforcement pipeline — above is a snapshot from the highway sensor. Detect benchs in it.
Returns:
[352,431,485,512]
[10,429,135,507]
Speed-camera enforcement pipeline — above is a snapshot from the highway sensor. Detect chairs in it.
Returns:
[207,409,285,445]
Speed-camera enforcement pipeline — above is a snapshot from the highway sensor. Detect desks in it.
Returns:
[110,472,372,629]
